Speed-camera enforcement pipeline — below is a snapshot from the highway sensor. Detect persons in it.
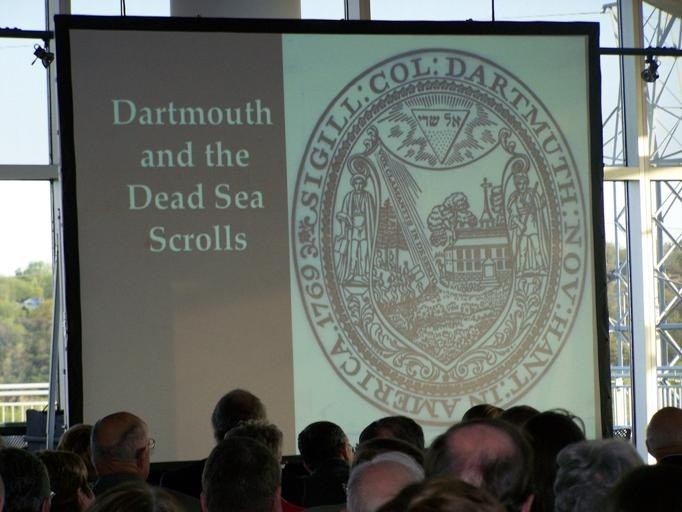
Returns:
[352,438,424,466]
[606,463,682,511]
[212,389,267,444]
[90,411,157,496]
[358,415,424,447]
[337,173,375,281]
[645,407,682,463]
[460,404,504,418]
[347,453,424,511]
[223,422,282,471]
[81,482,187,512]
[555,440,644,511]
[34,450,88,512]
[508,173,549,276]
[523,409,586,481]
[0,446,55,511]
[201,437,282,512]
[376,479,510,512]
[286,421,355,504]
[58,423,94,465]
[425,418,540,511]
[504,406,542,426]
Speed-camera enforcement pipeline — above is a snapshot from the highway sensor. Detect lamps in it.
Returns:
[642,59,661,82]
[31,44,54,68]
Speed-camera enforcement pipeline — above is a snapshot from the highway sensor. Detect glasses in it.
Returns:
[50,492,56,499]
[147,437,157,450]
